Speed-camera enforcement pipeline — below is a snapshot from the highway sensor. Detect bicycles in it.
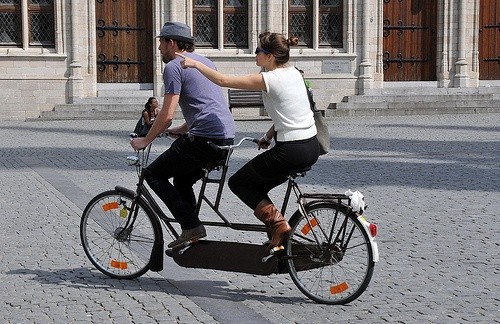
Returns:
[80,130,379,304]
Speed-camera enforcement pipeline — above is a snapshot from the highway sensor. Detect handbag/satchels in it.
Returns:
[295,67,330,155]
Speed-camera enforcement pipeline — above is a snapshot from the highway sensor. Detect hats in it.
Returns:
[153,22,194,42]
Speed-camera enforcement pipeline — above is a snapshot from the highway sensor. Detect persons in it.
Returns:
[131,21,234,248]
[134,97,171,135]
[176,32,321,251]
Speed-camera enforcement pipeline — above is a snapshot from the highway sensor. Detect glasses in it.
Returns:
[255,47,272,54]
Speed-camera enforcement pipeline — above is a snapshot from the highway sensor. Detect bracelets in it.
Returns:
[264,134,272,141]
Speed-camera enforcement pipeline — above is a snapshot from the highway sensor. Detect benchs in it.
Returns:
[228,88,268,116]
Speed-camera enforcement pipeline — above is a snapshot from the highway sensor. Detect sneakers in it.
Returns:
[168,223,207,248]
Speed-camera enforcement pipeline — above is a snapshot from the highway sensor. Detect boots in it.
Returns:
[253,199,292,251]
